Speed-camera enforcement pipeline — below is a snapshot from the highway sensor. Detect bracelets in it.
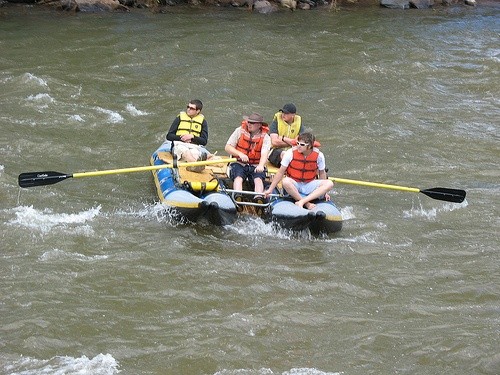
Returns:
[282,135,284,141]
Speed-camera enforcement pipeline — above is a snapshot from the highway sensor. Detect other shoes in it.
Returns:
[196,152,207,170]
[235,194,243,212]
[186,167,201,173]
[255,197,264,216]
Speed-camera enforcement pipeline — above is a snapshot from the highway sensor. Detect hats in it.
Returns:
[278,103,296,114]
[242,112,268,127]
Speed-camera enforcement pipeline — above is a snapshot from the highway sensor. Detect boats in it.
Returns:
[150,140,344,233]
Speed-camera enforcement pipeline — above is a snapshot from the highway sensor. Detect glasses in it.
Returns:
[297,141,310,147]
[187,106,196,110]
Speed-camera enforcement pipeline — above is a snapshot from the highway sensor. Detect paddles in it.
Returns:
[267,166,467,204]
[18,156,241,189]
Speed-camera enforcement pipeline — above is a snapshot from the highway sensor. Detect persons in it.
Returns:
[265,132,334,211]
[166,99,209,172]
[224,112,272,212]
[267,103,304,168]
